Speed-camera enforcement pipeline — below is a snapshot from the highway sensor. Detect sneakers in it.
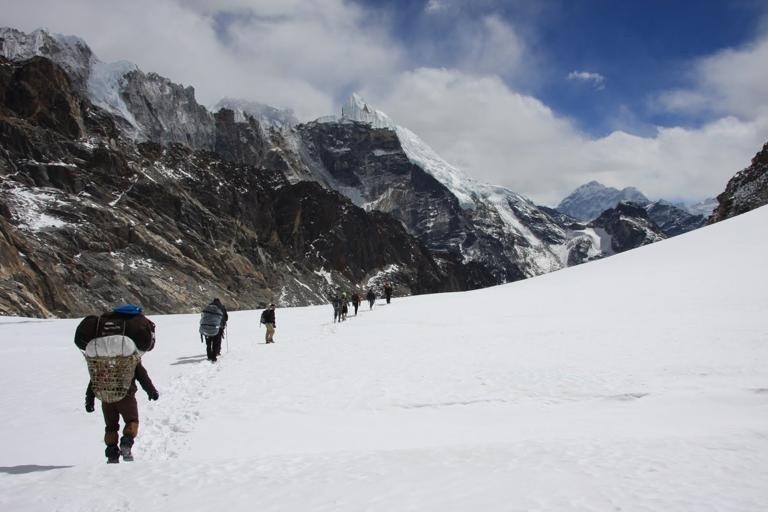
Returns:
[107,444,133,463]
[266,339,273,343]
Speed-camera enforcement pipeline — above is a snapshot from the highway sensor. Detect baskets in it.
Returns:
[86,354,138,403]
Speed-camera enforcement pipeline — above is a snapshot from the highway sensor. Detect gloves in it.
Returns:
[149,391,159,400]
[85,403,94,412]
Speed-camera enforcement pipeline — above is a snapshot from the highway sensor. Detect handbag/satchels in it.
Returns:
[75,311,155,351]
[199,304,222,336]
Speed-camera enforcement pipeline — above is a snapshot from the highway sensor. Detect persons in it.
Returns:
[352,291,361,315]
[264,303,277,345]
[85,352,159,464]
[204,297,230,362]
[384,282,393,304]
[340,291,351,320]
[332,295,344,323]
[366,288,376,310]
